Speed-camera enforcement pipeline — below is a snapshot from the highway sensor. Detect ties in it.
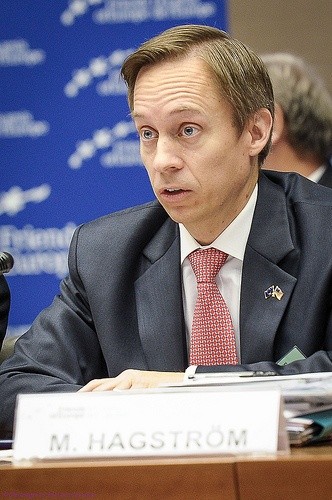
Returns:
[187,248,238,366]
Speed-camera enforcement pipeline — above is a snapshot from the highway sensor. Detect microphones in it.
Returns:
[0,251,13,275]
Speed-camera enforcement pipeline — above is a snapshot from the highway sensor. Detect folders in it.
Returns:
[286,409,332,446]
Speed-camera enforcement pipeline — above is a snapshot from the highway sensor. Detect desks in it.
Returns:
[1,438,331,499]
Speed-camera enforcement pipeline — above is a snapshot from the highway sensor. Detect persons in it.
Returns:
[0,23,332,452]
[243,53,332,191]
